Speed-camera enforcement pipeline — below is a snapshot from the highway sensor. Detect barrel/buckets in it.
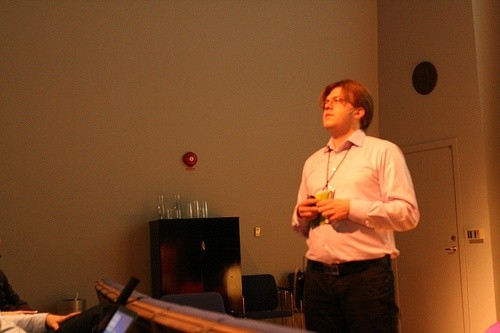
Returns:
[63,298,87,315]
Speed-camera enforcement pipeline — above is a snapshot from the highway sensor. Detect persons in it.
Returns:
[291,79,420,333]
[0,302,142,333]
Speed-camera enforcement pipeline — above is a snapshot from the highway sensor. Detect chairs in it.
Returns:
[241,273,309,330]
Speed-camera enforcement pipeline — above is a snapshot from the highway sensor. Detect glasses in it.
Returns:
[322,96,355,107]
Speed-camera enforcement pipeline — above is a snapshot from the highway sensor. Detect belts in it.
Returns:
[306,254,390,276]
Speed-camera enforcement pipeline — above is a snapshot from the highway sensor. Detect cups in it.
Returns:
[157,194,207,219]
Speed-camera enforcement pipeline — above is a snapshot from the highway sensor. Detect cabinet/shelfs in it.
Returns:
[148,217,243,323]
